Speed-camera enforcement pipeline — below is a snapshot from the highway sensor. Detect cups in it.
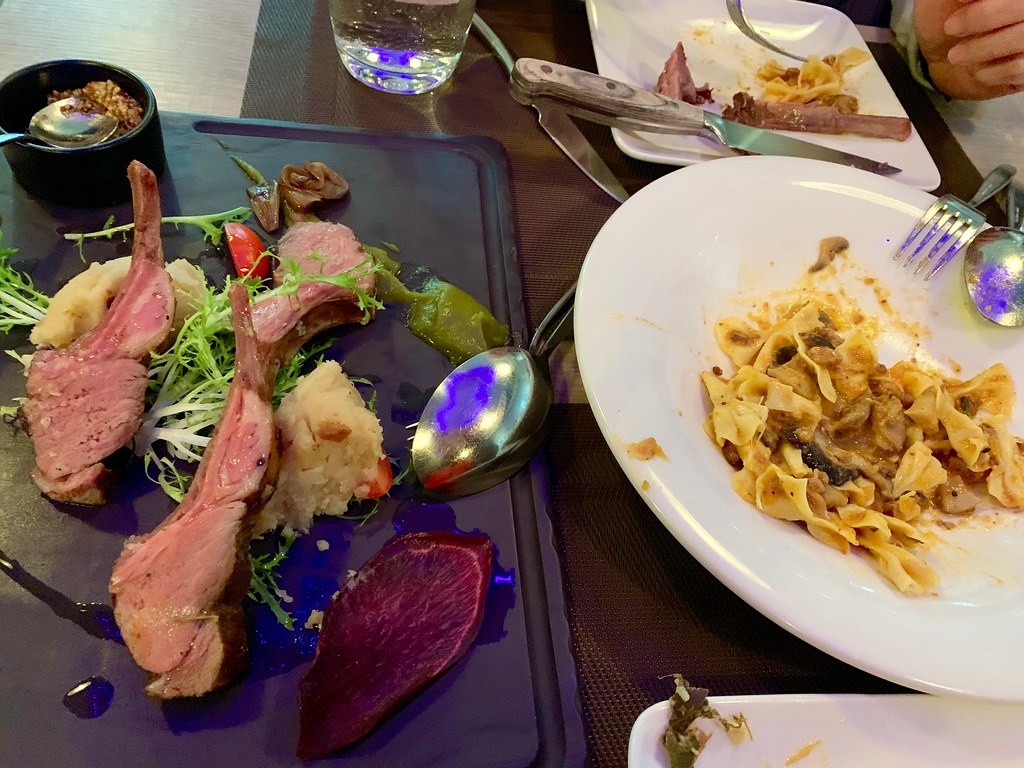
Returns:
[329,1,477,95]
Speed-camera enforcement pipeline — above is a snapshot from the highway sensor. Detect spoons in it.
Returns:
[411,306,574,498]
[1,98,119,149]
[965,179,1024,327]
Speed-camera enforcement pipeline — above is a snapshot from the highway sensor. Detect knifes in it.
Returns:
[471,13,629,204]
[509,58,902,177]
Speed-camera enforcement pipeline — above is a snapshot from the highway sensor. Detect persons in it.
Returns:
[802,0,1024,103]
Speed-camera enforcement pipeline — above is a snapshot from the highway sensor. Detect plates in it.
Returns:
[627,687,1024,768]
[574,155,1024,710]
[587,1,941,192]
[2,109,594,768]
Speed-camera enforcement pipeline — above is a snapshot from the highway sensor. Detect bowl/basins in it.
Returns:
[1,59,168,209]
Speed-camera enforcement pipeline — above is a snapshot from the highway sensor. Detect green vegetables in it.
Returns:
[0,206,386,630]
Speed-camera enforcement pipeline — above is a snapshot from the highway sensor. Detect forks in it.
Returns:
[894,164,1017,281]
